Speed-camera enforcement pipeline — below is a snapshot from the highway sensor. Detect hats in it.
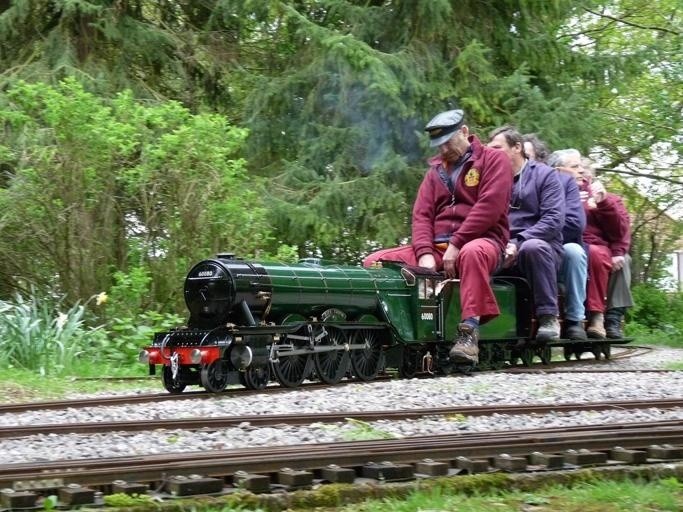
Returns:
[424,109,465,147]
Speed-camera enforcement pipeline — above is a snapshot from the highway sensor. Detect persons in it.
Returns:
[487,125,633,341]
[363,110,514,363]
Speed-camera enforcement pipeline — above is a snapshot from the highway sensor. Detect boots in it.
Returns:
[449,322,480,363]
[536,313,622,340]
